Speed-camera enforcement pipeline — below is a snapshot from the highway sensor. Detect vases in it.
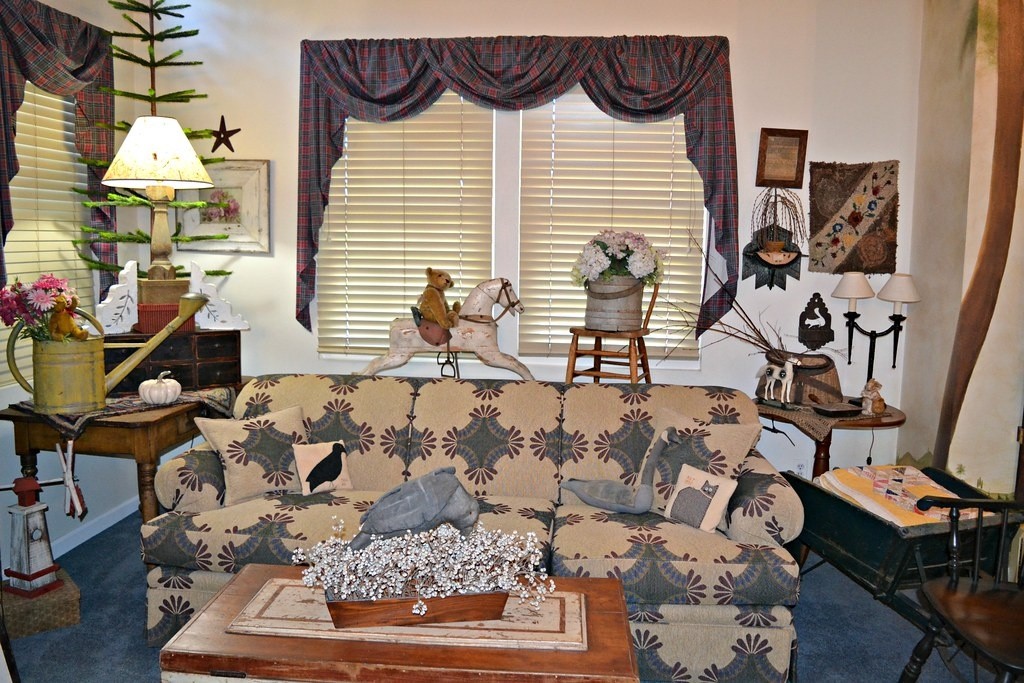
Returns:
[33,336,106,414]
[324,589,508,627]
[583,273,643,331]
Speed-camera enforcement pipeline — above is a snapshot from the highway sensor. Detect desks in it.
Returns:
[1,395,231,572]
[749,395,906,481]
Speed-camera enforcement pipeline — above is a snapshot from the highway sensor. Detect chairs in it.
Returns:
[563,284,659,383]
[897,416,1024,683]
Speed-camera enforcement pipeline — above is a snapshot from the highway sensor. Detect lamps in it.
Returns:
[101,116,215,303]
[828,272,921,409]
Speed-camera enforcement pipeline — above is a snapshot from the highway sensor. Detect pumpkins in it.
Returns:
[138,371,182,404]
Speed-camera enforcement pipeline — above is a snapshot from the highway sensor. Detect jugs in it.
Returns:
[6,293,209,415]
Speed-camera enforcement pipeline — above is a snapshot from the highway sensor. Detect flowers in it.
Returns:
[571,229,665,290]
[0,272,81,339]
[175,156,272,253]
[292,515,555,616]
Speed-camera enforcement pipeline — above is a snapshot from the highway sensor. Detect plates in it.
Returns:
[813,403,864,417]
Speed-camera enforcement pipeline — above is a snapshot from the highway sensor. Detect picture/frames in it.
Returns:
[755,128,809,188]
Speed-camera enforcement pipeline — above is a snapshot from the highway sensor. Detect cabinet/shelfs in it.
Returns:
[103,326,242,397]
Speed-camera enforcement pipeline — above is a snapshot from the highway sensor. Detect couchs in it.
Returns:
[138,372,804,682]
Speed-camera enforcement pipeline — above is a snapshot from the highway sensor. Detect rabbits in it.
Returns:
[861,377,887,415]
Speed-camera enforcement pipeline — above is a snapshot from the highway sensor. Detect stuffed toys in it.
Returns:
[562,427,682,514]
[349,466,479,551]
[421,267,461,328]
[47,293,89,342]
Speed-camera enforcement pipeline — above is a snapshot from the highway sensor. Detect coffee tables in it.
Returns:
[160,564,642,683]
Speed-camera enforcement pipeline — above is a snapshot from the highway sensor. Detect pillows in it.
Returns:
[293,439,352,497]
[666,463,738,534]
[630,408,762,516]
[194,406,308,508]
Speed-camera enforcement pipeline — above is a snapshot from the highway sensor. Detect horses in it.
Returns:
[755,357,801,405]
[349,278,535,381]
